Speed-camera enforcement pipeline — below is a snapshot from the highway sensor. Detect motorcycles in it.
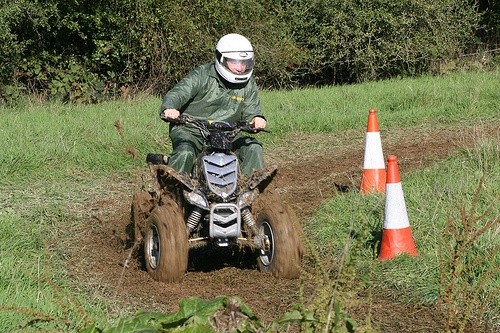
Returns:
[131,109,304,282]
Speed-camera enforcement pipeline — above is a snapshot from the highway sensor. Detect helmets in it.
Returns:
[215,33,255,84]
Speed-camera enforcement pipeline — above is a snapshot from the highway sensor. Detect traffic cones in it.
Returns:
[377,153,417,259]
[359,109,386,195]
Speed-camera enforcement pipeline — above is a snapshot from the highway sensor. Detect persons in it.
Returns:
[159,33,267,180]
